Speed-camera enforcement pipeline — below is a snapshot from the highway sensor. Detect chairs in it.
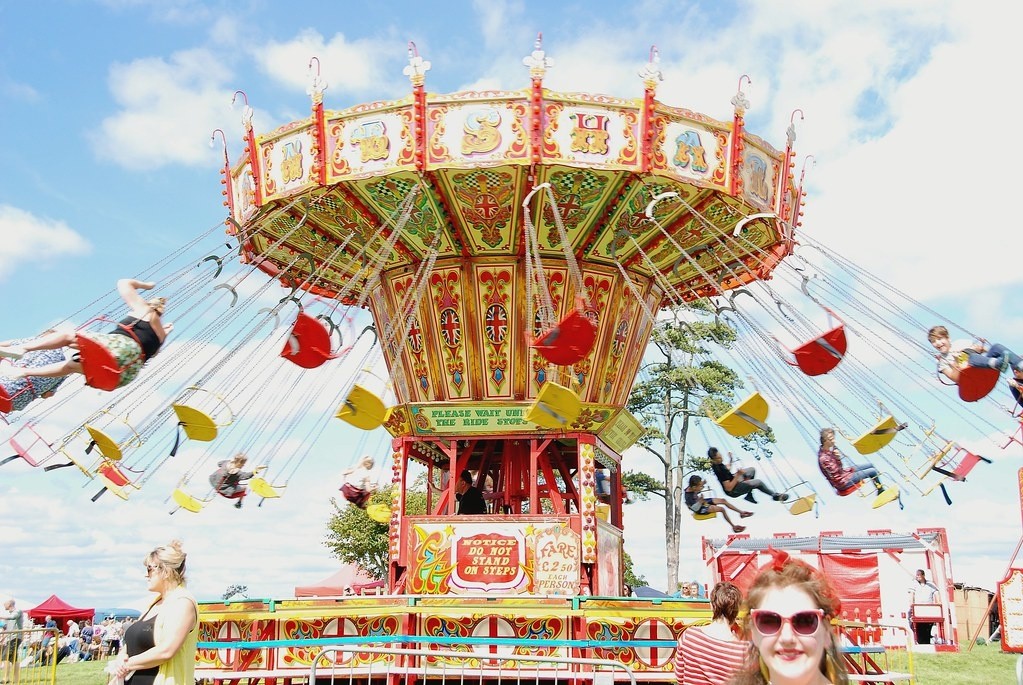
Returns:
[92,636,114,661]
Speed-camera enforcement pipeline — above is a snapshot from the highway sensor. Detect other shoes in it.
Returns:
[235,501,241,509]
[734,526,745,533]
[745,493,757,503]
[0,346,25,358]
[773,493,788,501]
[0,365,19,380]
[740,511,753,517]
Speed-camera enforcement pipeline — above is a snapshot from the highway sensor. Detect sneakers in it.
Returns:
[995,350,1009,372]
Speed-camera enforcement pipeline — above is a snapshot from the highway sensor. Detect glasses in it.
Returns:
[623,588,627,590]
[750,609,824,636]
[683,587,688,589]
[148,566,162,573]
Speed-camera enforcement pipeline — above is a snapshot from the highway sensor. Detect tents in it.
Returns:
[93,607,142,626]
[22,595,96,636]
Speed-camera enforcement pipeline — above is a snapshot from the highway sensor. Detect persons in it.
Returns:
[685,475,754,534]
[0,599,23,685]
[103,538,200,685]
[595,468,635,504]
[217,453,268,508]
[908,568,942,644]
[426,470,488,515]
[0,278,175,412]
[709,446,789,504]
[0,612,132,667]
[343,456,375,508]
[818,429,887,495]
[747,556,850,685]
[674,581,765,685]
[927,325,1023,409]
